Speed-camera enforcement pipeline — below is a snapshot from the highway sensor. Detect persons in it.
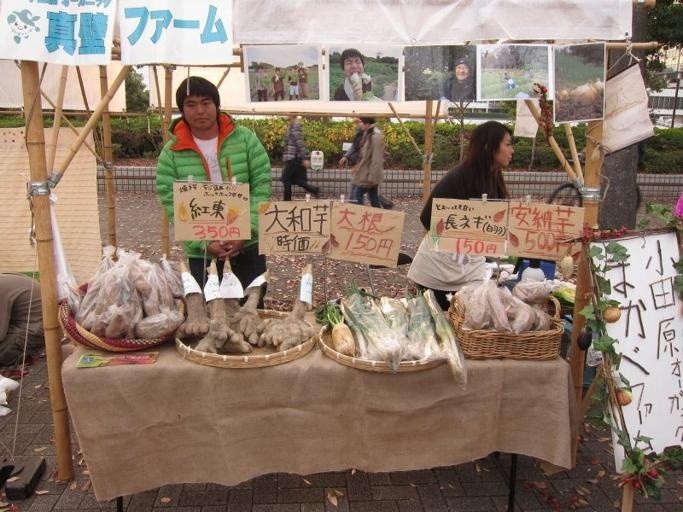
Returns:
[442,57,474,102]
[286,75,299,100]
[298,68,309,100]
[256,66,268,101]
[155,76,272,312]
[337,117,385,208]
[279,116,319,200]
[0,273,45,366]
[406,121,515,311]
[271,67,284,101]
[334,48,372,100]
[501,72,515,90]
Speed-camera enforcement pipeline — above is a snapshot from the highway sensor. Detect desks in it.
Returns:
[59,312,584,511]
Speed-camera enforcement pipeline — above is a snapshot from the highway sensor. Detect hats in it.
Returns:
[454,55,472,70]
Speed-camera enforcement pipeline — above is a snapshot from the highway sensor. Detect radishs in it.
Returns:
[331,321,356,357]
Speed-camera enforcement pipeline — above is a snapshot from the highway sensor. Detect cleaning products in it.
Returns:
[522,258,546,283]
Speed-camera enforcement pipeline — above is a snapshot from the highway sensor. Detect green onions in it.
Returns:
[340,279,465,384]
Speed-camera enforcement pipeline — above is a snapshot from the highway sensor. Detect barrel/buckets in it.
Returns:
[311,151,324,171]
